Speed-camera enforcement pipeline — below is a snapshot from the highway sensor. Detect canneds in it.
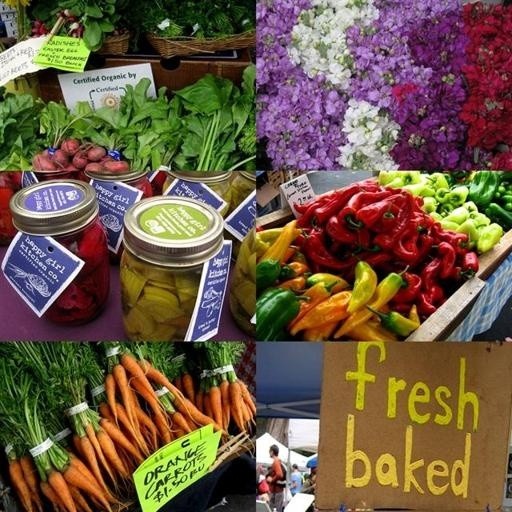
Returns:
[0,171,256,343]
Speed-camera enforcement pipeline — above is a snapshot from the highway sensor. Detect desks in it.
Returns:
[0,446,256,512]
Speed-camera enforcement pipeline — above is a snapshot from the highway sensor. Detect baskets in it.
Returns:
[93,32,134,55]
[144,31,256,60]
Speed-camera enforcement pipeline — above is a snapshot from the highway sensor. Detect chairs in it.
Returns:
[255,500,273,512]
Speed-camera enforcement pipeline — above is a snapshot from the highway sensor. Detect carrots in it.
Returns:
[34,138,129,175]
[9,352,256,512]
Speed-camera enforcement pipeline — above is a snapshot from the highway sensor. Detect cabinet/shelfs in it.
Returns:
[1,0,258,171]
[255,204,512,342]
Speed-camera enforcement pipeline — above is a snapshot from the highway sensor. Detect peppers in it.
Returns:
[256,170,512,341]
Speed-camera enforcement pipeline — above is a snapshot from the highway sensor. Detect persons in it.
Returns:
[265,444,286,511]
[256,462,316,498]
